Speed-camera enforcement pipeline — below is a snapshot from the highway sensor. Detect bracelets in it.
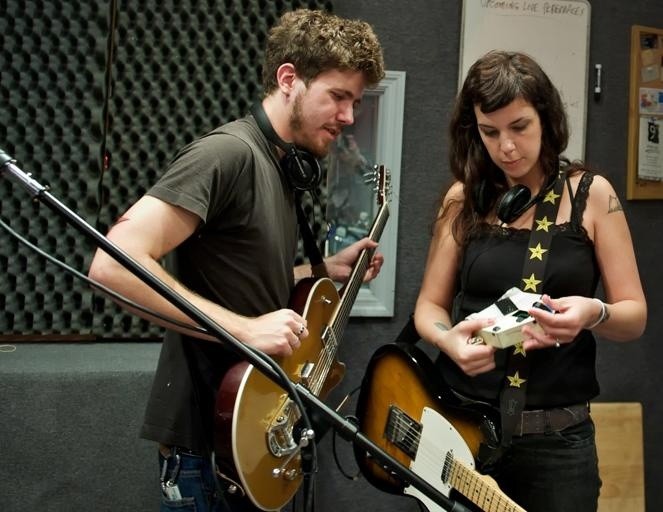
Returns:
[586,296,606,329]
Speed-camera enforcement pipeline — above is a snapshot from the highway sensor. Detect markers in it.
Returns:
[594,64,602,102]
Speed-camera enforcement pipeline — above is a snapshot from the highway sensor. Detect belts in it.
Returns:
[453,390,591,434]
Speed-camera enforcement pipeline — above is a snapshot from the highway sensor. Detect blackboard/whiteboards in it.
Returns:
[456,0,591,164]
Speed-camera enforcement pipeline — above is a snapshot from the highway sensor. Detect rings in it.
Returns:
[554,337,561,348]
[297,326,305,334]
[292,336,299,344]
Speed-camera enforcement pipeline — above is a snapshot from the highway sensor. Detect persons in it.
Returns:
[87,4,386,511]
[410,55,647,511]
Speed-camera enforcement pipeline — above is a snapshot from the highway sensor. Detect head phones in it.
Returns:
[248,99,324,191]
[494,175,561,224]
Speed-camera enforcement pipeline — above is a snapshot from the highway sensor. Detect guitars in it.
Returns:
[354,343,527,511]
[211,165,392,511]
